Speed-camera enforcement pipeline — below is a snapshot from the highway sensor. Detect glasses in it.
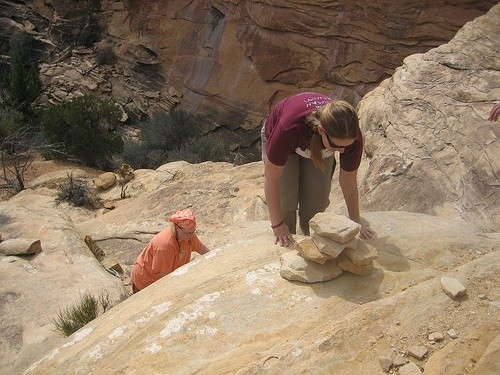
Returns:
[324,132,354,149]
[182,230,197,236]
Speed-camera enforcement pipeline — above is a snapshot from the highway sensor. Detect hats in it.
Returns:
[168,208,196,229]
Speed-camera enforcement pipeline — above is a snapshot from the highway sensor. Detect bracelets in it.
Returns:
[270,220,284,228]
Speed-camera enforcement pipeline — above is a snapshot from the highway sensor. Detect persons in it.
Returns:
[259,92,375,247]
[131,208,211,294]
